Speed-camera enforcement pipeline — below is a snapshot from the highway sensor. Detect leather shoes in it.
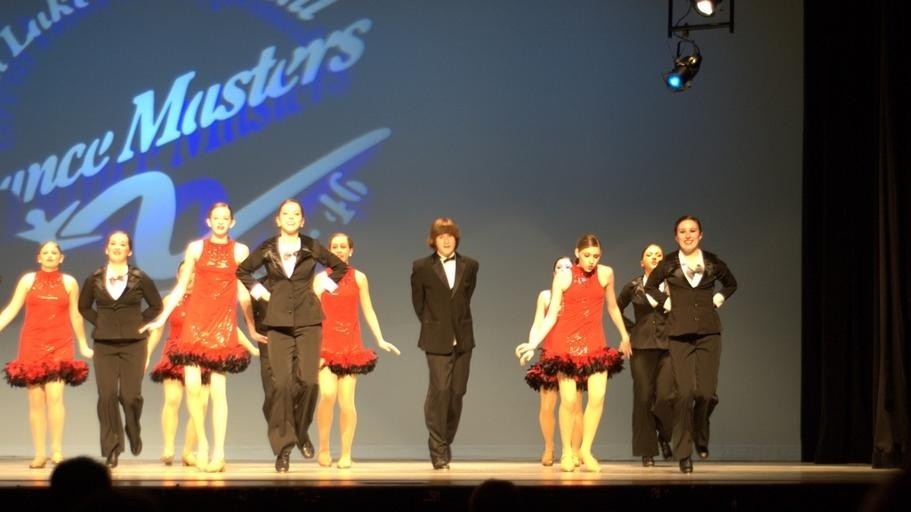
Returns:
[302,441,314,459]
[439,464,450,469]
[660,442,672,462]
[275,455,289,473]
[698,450,708,459]
[105,451,120,468]
[642,456,655,467]
[130,441,142,455]
[680,456,693,473]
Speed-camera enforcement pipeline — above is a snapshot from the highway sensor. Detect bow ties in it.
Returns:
[284,251,299,259]
[444,255,456,263]
[687,264,703,280]
[109,275,123,283]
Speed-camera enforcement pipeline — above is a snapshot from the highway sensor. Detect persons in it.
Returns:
[410,217,480,470]
[0,241,94,468]
[519,256,581,466]
[138,202,268,472]
[78,230,164,468]
[236,199,347,473]
[643,215,738,472]
[617,243,673,466]
[313,232,401,469]
[144,259,260,465]
[49,457,112,511]
[514,234,633,472]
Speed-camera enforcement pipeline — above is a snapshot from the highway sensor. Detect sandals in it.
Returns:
[160,455,174,466]
[181,456,196,467]
[195,461,208,471]
[337,458,352,469]
[542,451,554,466]
[585,459,601,472]
[561,456,575,472]
[206,459,226,473]
[50,457,63,464]
[29,459,46,468]
[318,450,331,467]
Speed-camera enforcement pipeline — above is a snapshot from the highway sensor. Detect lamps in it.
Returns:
[662,36,704,94]
[690,0,722,17]
